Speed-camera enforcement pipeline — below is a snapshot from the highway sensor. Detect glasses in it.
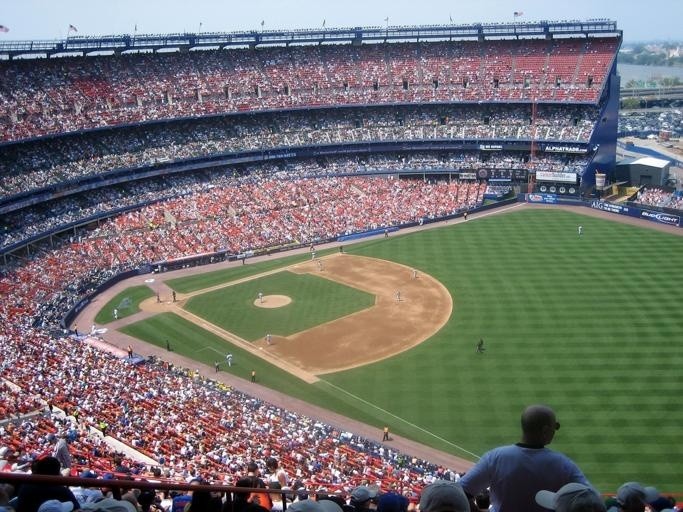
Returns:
[555,422,560,430]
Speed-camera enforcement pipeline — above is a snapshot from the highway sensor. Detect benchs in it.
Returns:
[0,40,619,504]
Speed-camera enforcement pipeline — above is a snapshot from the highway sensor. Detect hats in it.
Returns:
[0,433,683,512]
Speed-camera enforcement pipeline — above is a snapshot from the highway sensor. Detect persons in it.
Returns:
[0,37,682,512]
